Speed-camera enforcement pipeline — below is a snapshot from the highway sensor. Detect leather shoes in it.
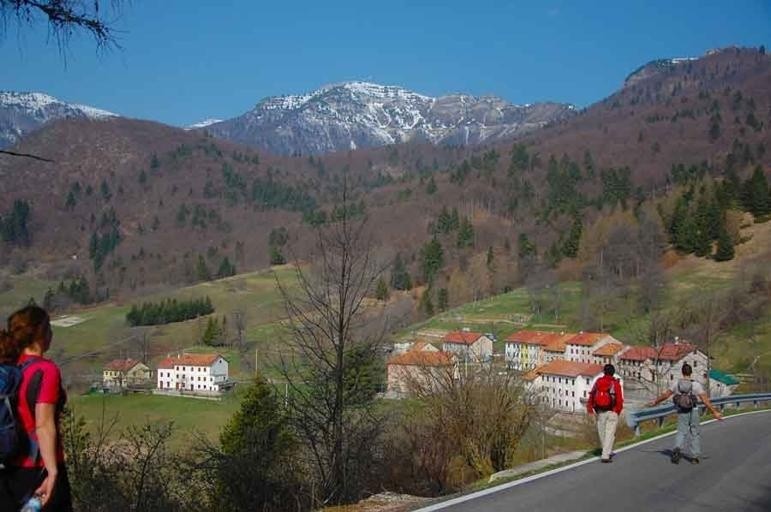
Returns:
[601,459,613,463]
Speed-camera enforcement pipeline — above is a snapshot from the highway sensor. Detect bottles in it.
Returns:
[20,495,45,512]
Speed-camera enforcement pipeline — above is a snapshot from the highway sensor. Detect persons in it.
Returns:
[0,306,72,512]
[587,364,624,463]
[645,365,725,465]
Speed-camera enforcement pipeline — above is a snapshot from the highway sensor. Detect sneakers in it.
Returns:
[671,447,681,464]
[692,458,698,464]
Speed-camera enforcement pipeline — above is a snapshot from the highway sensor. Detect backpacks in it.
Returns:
[0,355,46,467]
[594,379,616,410]
[673,382,696,410]
